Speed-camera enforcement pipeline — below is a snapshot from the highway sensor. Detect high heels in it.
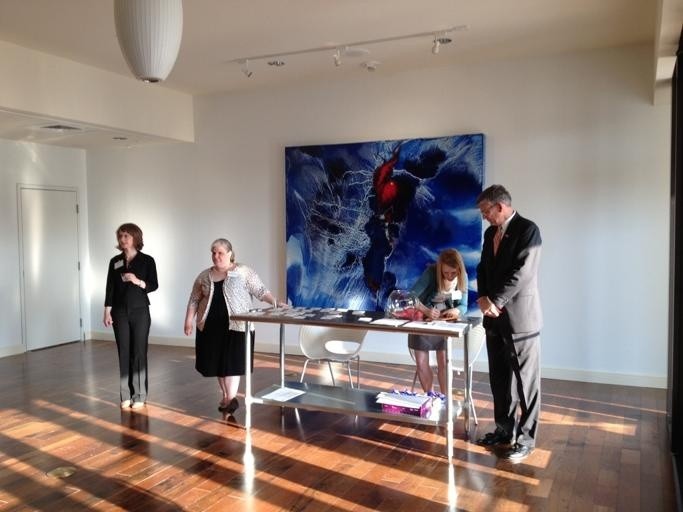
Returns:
[218,398,239,414]
[132,400,146,409]
[120,399,133,409]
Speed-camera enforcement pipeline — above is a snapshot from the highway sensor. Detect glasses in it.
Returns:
[481,203,497,214]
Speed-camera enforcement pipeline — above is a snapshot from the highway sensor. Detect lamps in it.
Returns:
[113,0,183,84]
[219,25,468,79]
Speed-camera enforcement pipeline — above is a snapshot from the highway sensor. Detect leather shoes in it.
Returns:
[502,442,535,461]
[476,430,513,446]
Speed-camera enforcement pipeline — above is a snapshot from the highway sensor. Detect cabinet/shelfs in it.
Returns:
[230,307,482,460]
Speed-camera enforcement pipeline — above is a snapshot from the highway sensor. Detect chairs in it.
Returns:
[299,326,368,389]
[409,325,486,425]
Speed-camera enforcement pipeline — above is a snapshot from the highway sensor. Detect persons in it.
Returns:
[407,246,471,397]
[182,237,288,415]
[102,222,158,409]
[474,183,543,464]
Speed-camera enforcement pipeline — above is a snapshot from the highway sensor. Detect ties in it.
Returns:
[492,226,501,256]
